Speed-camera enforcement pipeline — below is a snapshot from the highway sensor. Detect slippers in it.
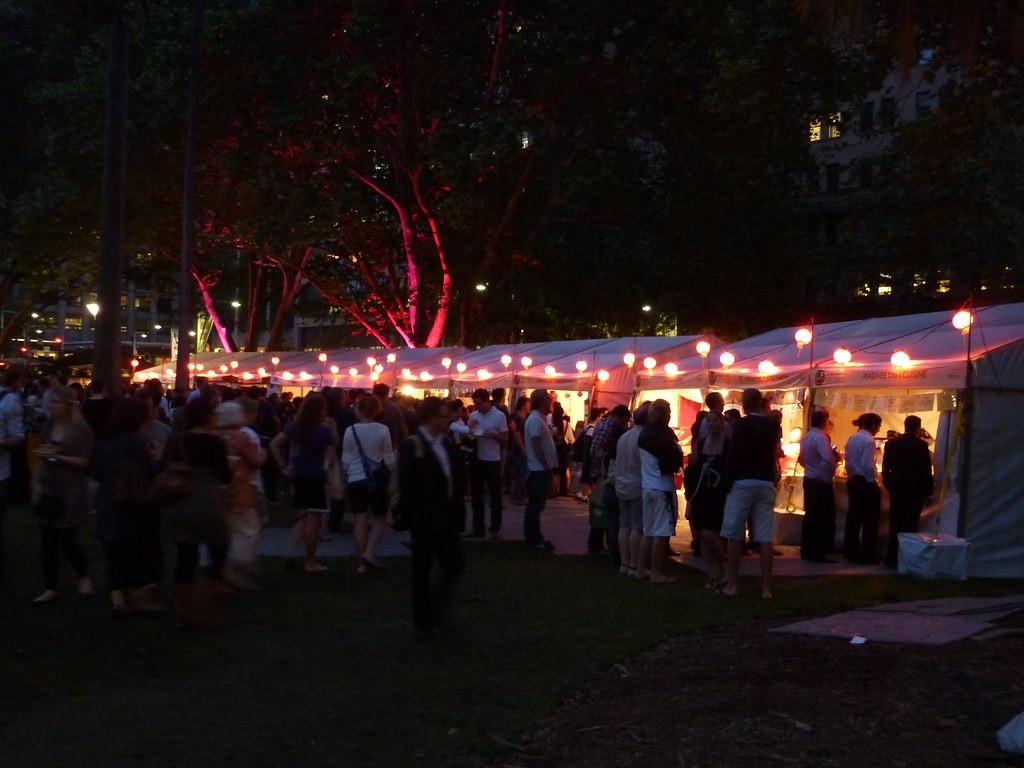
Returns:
[634,568,679,586]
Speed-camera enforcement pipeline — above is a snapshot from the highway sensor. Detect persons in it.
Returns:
[0,364,936,643]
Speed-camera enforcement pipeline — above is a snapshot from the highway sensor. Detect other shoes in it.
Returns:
[461,526,503,540]
[586,546,611,561]
[799,546,837,566]
[354,551,389,574]
[109,602,130,618]
[282,553,332,574]
[79,579,95,598]
[722,585,740,598]
[760,592,777,602]
[28,590,61,608]
[525,538,557,551]
[703,572,727,591]
[845,549,884,566]
[415,620,438,641]
[619,561,644,577]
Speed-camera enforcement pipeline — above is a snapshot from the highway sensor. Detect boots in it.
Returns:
[178,571,231,634]
[172,576,195,631]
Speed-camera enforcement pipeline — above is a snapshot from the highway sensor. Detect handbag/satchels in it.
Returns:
[572,425,598,464]
[587,479,609,531]
[362,457,392,489]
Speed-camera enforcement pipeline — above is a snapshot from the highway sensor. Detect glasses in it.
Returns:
[431,413,449,421]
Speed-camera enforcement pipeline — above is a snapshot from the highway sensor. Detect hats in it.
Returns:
[214,401,246,430]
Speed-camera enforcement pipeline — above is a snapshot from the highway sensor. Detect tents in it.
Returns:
[132,303,1024,579]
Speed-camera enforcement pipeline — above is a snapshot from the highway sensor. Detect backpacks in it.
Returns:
[380,436,424,533]
